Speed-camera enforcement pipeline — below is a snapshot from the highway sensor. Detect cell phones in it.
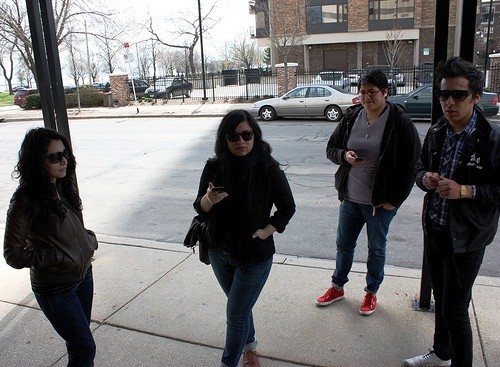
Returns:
[212,186,224,193]
[356,157,365,160]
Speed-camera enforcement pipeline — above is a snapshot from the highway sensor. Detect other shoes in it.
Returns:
[243,350,260,367]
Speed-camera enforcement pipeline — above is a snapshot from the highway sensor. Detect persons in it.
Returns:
[3,128,98,367]
[315,68,422,315]
[193,110,296,367]
[402,56,500,367]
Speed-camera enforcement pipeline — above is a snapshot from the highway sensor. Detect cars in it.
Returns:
[415,74,433,89]
[345,72,361,85]
[129,79,150,101]
[144,77,193,100]
[249,85,361,122]
[386,68,405,86]
[93,82,111,92]
[313,71,350,93]
[13,89,37,108]
[386,83,500,118]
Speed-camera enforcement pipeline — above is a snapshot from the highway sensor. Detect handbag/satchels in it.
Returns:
[183,214,210,265]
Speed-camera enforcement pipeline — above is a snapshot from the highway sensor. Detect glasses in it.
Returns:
[46,148,69,164]
[227,131,253,142]
[358,88,384,97]
[433,89,474,100]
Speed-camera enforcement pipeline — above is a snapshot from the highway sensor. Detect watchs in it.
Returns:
[461,185,467,198]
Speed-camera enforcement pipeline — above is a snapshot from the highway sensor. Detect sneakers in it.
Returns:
[404,351,451,367]
[315,287,345,306]
[359,294,377,315]
